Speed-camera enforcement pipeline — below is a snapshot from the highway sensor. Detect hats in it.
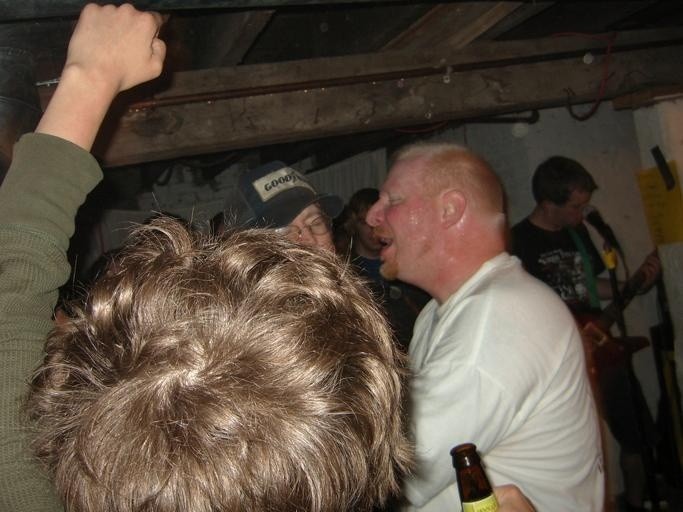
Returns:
[223,160,345,231]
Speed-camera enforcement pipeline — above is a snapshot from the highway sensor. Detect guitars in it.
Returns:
[566,247,659,373]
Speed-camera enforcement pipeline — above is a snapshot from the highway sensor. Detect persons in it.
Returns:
[1,3,538,512]
[512,152,663,510]
[364,142,605,512]
[91,159,423,352]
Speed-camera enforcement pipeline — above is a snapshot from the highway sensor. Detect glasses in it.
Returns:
[274,211,332,245]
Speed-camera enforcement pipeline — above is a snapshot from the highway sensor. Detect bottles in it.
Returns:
[449,441,500,512]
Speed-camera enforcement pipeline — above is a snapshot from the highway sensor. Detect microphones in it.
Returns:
[582,205,624,258]
